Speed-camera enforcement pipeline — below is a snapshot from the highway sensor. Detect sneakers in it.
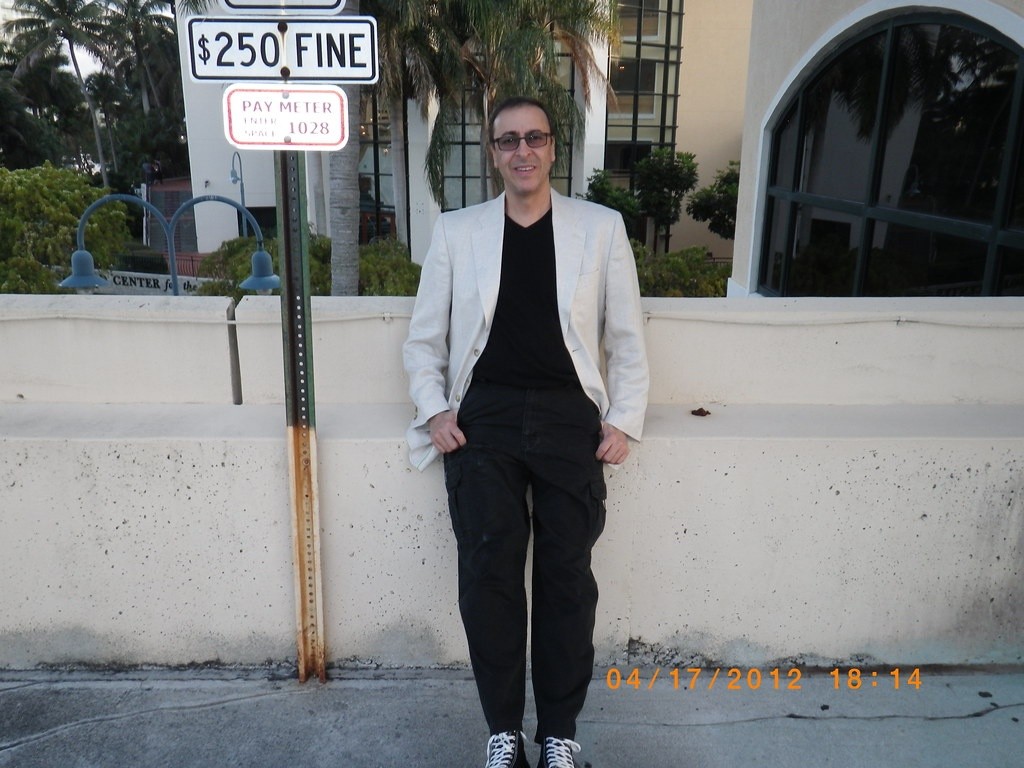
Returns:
[485,730,531,768]
[537,736,582,768]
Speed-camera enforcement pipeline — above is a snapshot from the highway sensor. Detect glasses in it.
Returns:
[492,132,552,152]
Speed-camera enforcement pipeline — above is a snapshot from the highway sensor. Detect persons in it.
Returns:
[403,97,649,768]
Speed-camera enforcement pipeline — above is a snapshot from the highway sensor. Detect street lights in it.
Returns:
[52,195,284,301]
[229,151,250,238]
